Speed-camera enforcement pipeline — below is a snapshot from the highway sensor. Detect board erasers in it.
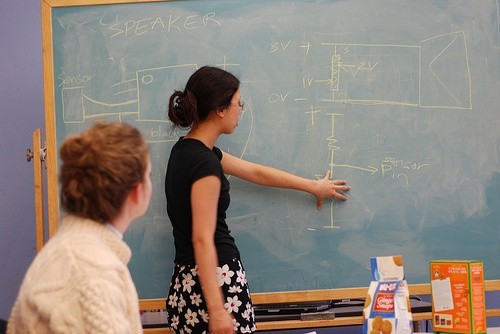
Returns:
[300,311,335,321]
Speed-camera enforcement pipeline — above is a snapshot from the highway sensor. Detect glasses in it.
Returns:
[224,99,245,110]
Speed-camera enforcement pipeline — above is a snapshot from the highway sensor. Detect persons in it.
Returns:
[164,65,352,334]
[5,121,153,334]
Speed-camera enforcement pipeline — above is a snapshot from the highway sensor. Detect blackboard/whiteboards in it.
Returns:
[39,0,500,315]
